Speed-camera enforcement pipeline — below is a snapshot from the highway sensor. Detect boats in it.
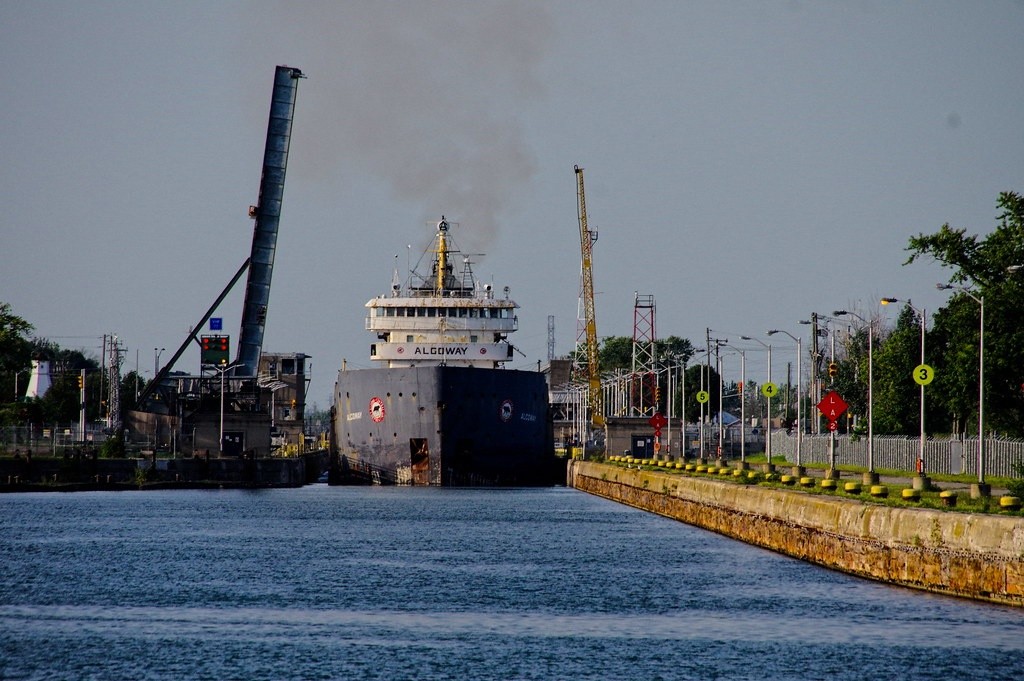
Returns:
[332,215,558,488]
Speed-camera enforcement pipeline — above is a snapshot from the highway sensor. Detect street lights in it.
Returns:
[935,282,985,483]
[880,298,926,478]
[766,330,801,465]
[697,349,722,458]
[798,320,835,469]
[717,343,745,462]
[832,310,873,473]
[678,349,704,459]
[739,336,771,464]
